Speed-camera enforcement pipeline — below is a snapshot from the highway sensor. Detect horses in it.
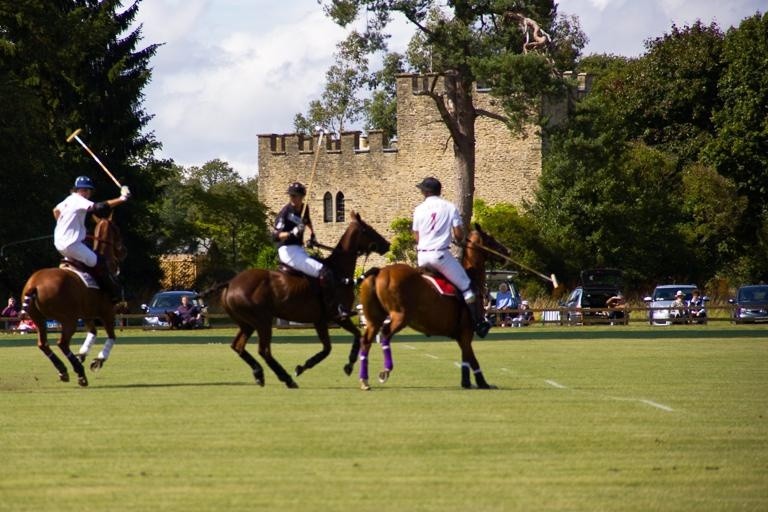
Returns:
[20,212,126,387]
[186,209,390,388]
[354,222,508,391]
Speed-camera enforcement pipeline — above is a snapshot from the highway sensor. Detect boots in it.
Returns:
[319,266,359,318]
[466,299,491,338]
[86,250,121,302]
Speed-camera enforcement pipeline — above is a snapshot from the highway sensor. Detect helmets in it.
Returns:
[415,177,442,195]
[74,175,96,191]
[284,182,306,197]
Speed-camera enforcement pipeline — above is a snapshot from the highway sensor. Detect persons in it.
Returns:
[607,293,631,325]
[16,319,37,334]
[685,290,708,325]
[1,297,18,328]
[272,183,357,322]
[411,177,489,337]
[510,301,535,327]
[668,290,689,324]
[480,286,495,326]
[175,296,202,328]
[50,176,134,298]
[497,281,516,325]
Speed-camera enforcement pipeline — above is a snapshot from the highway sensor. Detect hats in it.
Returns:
[675,290,686,298]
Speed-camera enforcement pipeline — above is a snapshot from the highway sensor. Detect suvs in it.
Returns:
[138,290,210,330]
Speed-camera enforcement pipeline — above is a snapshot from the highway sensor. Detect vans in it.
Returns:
[726,283,767,326]
[559,267,630,325]
[477,267,524,326]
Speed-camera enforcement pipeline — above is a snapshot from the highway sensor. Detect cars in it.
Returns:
[642,282,710,327]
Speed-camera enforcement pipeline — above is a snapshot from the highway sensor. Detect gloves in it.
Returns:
[118,186,132,201]
[290,223,304,238]
